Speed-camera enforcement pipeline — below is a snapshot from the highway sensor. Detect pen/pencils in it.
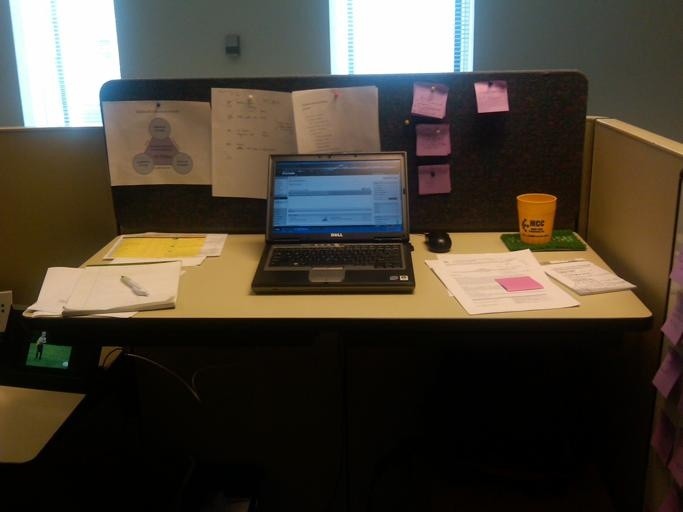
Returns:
[121,275,150,297]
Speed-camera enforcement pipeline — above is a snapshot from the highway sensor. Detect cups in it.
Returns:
[514,192,559,243]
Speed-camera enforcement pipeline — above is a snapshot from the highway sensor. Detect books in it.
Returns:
[544,257,637,295]
[61,259,182,316]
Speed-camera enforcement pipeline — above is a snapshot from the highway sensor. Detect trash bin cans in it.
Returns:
[172,462,266,511]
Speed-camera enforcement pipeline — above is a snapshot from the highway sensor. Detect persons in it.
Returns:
[33,331,46,360]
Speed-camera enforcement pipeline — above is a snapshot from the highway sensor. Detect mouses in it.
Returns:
[425,231,452,253]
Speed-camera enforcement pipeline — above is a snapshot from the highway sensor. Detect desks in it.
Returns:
[20,229,654,511]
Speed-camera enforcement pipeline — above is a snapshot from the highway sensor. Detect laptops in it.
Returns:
[252,152,415,294]
